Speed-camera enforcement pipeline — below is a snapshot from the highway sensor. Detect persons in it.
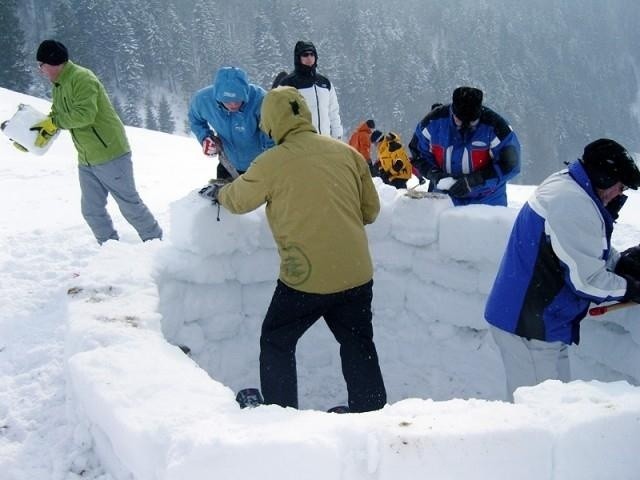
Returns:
[482,138,640,404]
[349,119,378,177]
[197,84,388,415]
[276,40,343,141]
[1,39,164,245]
[370,130,413,189]
[187,66,274,182]
[408,86,522,207]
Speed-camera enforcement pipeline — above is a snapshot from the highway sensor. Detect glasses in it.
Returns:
[300,51,314,57]
[37,62,44,68]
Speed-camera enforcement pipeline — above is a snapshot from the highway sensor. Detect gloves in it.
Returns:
[9,137,28,154]
[30,117,58,147]
[200,134,222,157]
[449,170,483,197]
[426,164,446,189]
[620,275,640,304]
[199,185,222,205]
[614,257,640,276]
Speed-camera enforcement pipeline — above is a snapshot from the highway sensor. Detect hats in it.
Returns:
[370,130,384,144]
[35,39,69,65]
[366,119,375,129]
[451,87,483,121]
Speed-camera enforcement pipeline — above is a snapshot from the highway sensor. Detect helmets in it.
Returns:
[581,139,640,191]
